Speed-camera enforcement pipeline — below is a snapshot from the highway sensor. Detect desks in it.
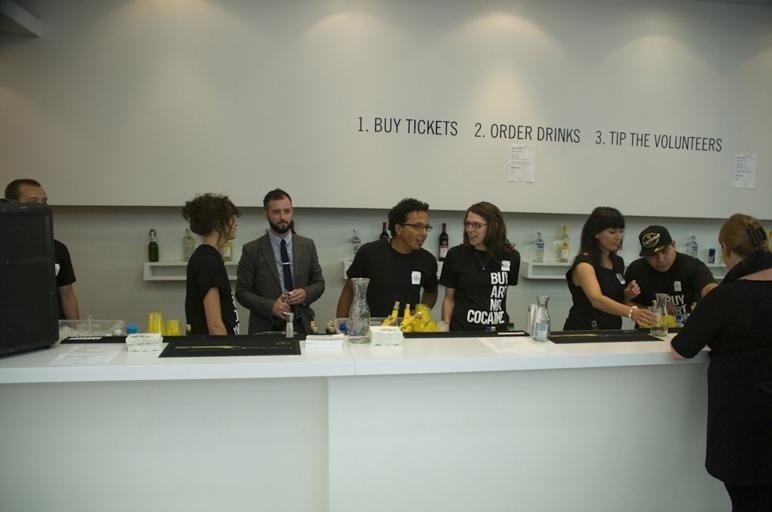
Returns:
[0,330,772,510]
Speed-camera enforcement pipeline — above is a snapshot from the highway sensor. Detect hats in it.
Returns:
[639,225,672,256]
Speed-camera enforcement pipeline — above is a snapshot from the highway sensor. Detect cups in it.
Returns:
[147,312,163,336]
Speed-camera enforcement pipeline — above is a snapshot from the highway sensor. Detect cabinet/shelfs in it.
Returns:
[343,256,446,282]
[142,261,240,283]
[707,262,727,280]
[521,258,573,281]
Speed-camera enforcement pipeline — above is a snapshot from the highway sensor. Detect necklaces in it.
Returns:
[473,247,491,271]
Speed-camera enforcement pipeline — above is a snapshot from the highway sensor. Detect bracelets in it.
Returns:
[628,305,638,320]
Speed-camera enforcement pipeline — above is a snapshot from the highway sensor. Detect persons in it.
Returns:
[563,206,657,330]
[669,214,772,511]
[336,197,438,319]
[234,187,325,335]
[182,192,241,336]
[5,179,80,320]
[624,224,719,330]
[438,201,520,331]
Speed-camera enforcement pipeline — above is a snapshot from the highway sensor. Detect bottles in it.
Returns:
[708,249,715,264]
[182,229,195,262]
[220,240,232,262]
[351,229,362,260]
[688,235,698,259]
[535,232,544,264]
[559,225,569,262]
[166,320,182,336]
[148,229,159,262]
[438,223,448,261]
[380,223,389,241]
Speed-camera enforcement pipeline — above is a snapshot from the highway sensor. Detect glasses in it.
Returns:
[404,224,432,232]
[466,222,487,228]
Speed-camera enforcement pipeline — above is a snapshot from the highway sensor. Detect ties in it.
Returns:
[280,239,296,313]
